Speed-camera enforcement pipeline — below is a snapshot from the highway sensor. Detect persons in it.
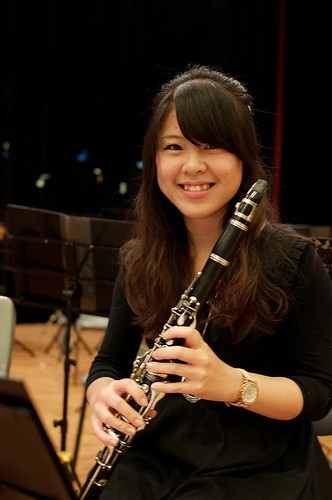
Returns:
[86,68,332,499]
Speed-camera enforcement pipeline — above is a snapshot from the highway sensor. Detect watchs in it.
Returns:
[227,367,258,409]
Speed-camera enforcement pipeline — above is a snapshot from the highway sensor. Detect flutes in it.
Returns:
[77,179,266,500]
